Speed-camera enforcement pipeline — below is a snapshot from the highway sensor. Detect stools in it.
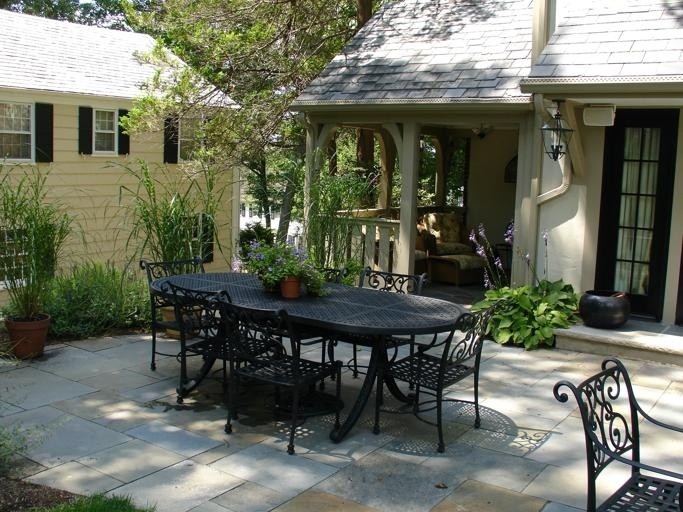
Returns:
[431,254,482,289]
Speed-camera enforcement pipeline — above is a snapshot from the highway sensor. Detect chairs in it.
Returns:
[293,266,351,390]
[327,266,427,407]
[418,192,474,255]
[216,298,343,456]
[137,255,235,384]
[552,356,683,512]
[366,206,432,287]
[159,278,280,422]
[371,298,505,453]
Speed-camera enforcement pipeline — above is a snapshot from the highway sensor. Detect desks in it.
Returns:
[149,271,465,445]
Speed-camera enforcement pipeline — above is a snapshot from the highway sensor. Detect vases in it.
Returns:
[279,277,300,298]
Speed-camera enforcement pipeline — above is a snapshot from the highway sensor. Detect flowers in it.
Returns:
[235,226,332,297]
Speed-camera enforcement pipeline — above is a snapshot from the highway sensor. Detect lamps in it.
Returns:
[419,133,426,152]
[471,120,494,140]
[539,99,574,162]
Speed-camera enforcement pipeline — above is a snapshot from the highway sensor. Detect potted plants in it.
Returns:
[0,151,96,358]
[100,152,249,341]
